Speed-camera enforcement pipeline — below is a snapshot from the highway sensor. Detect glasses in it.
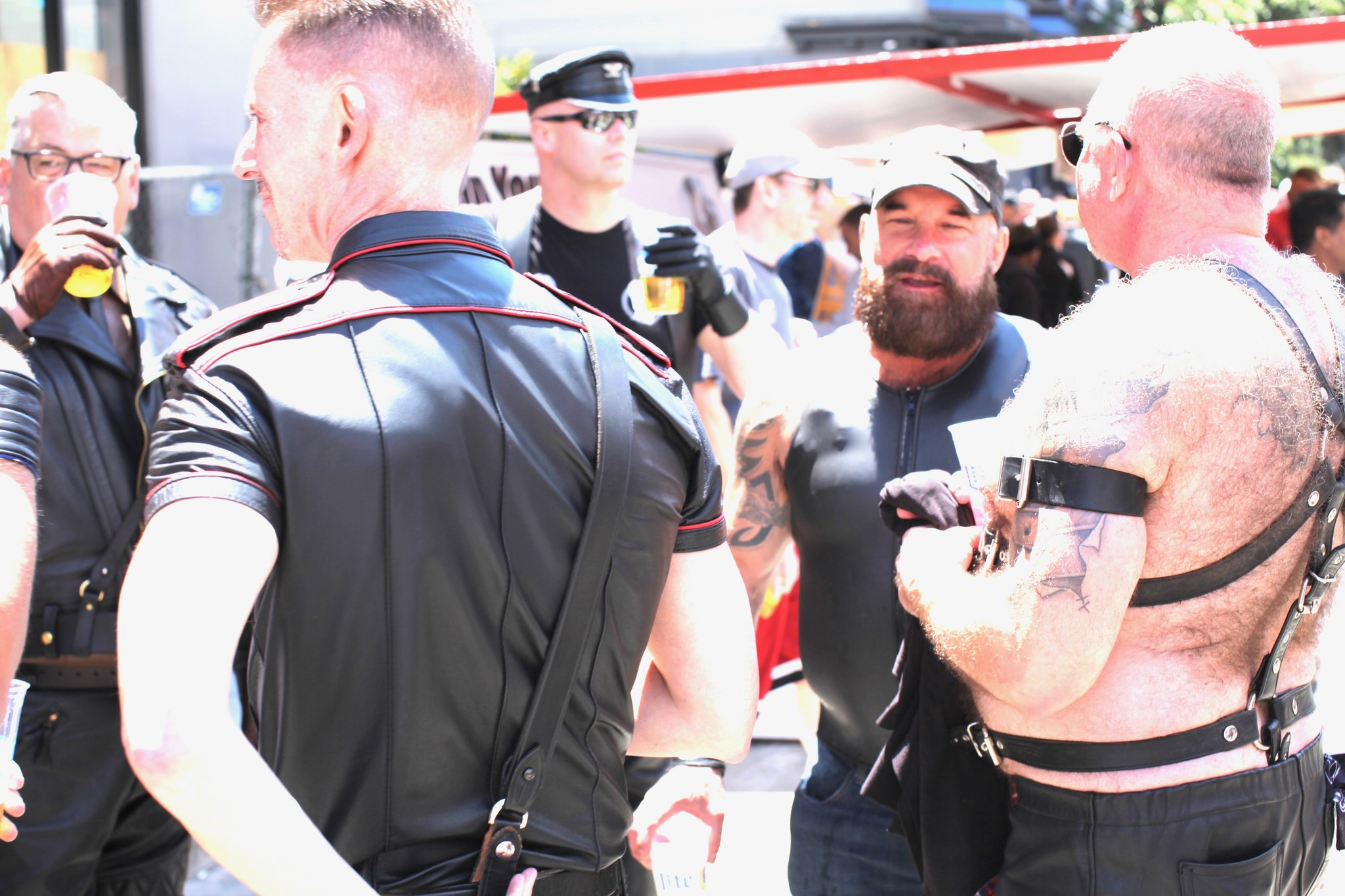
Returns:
[10,147,136,185]
[531,107,638,133]
[780,172,820,193]
[1059,118,1131,169]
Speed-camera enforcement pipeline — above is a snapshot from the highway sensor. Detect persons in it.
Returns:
[0,0,1345,896]
[629,124,1052,896]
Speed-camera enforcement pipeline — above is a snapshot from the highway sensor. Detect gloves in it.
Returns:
[8,212,126,322]
[641,221,749,335]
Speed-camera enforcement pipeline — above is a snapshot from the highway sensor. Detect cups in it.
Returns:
[45,172,118,298]
[635,214,697,315]
[946,416,997,524]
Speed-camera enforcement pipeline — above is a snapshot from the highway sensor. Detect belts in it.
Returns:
[25,610,119,657]
[949,677,1323,772]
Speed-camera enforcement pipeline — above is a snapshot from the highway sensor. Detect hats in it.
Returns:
[722,124,846,188]
[519,45,641,113]
[869,123,1007,228]
